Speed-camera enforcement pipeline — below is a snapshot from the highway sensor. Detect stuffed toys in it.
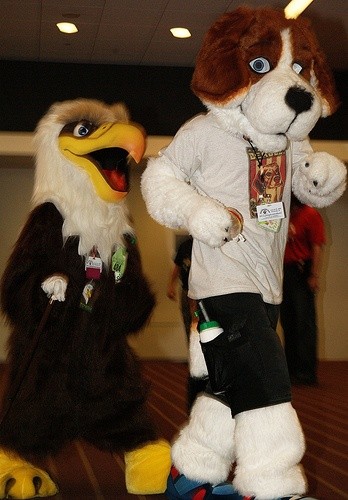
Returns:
[0,100,174,500]
[140,6,348,500]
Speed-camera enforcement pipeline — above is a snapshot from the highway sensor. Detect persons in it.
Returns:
[166,235,194,341]
[280,188,326,386]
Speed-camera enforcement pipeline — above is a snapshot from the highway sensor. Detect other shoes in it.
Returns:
[292,374,318,386]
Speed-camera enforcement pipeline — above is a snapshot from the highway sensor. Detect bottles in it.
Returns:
[199,320,223,344]
